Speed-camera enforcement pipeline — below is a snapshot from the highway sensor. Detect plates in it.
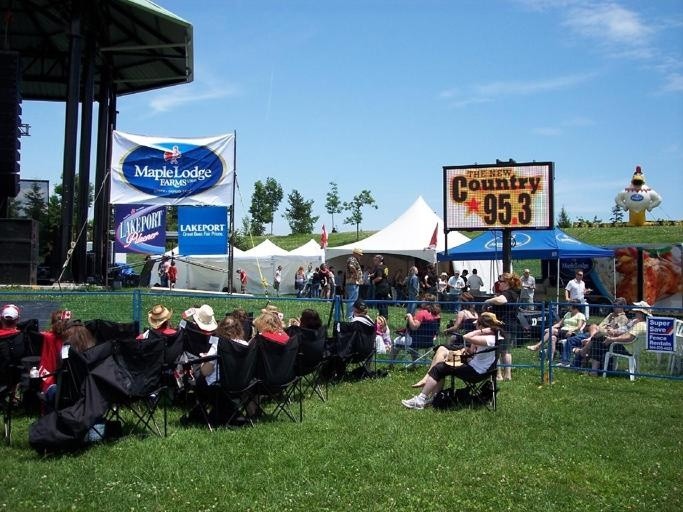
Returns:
[522,285,530,287]
[585,290,592,292]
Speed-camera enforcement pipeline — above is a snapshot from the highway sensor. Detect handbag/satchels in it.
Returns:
[393,333,412,346]
[432,390,454,408]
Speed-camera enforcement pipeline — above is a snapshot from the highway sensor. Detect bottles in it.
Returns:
[30,366,38,382]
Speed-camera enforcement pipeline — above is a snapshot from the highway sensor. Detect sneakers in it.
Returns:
[558,363,570,367]
[401,392,437,410]
[40,392,47,401]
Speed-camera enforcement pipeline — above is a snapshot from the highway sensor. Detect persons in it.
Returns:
[0,296,378,429]
[564,269,592,322]
[483,274,522,383]
[443,292,479,345]
[289,261,535,312]
[372,316,391,353]
[368,252,389,322]
[385,293,442,370]
[573,301,653,377]
[157,255,284,297]
[555,296,629,369]
[525,298,583,367]
[342,249,363,319]
[399,309,500,411]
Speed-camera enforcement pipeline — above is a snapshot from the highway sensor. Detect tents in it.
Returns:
[324,196,513,297]
[149,242,243,291]
[239,238,325,295]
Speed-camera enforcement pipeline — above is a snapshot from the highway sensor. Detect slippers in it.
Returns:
[584,372,598,376]
[573,348,580,356]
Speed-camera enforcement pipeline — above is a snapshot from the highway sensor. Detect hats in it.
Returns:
[440,272,449,278]
[457,292,475,302]
[354,299,366,309]
[0,303,23,324]
[444,350,464,367]
[463,270,469,274]
[192,304,217,332]
[146,304,173,329]
[613,298,625,305]
[631,300,653,318]
[472,312,504,325]
[260,305,278,315]
[352,248,363,256]
[455,270,460,275]
[57,319,83,336]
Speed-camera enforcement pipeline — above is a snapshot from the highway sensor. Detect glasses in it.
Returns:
[498,279,505,283]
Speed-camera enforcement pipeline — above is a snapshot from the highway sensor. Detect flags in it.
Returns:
[104,128,236,207]
